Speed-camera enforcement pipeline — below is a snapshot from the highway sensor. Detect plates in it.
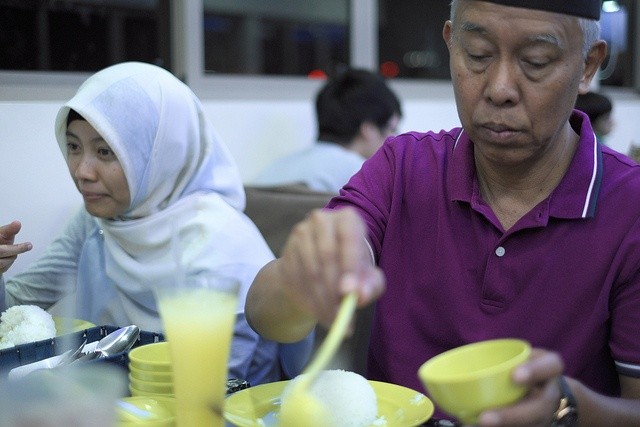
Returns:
[55,317,94,337]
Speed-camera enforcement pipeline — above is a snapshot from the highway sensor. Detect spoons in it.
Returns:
[280,292,358,427]
[68,324,140,364]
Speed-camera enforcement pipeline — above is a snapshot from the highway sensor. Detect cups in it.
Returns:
[148,275,242,427]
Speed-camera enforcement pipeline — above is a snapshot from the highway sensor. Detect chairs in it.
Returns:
[240,182,341,259]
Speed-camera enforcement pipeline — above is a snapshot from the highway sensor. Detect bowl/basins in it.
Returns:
[223,378,434,427]
[118,395,177,426]
[128,341,173,395]
[416,337,531,426]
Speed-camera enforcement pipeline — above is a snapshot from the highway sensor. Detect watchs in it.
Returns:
[553,376,578,427]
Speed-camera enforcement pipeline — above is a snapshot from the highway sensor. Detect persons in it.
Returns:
[243,3,639,426]
[262,68,402,193]
[1,60,280,380]
[573,91,613,147]
